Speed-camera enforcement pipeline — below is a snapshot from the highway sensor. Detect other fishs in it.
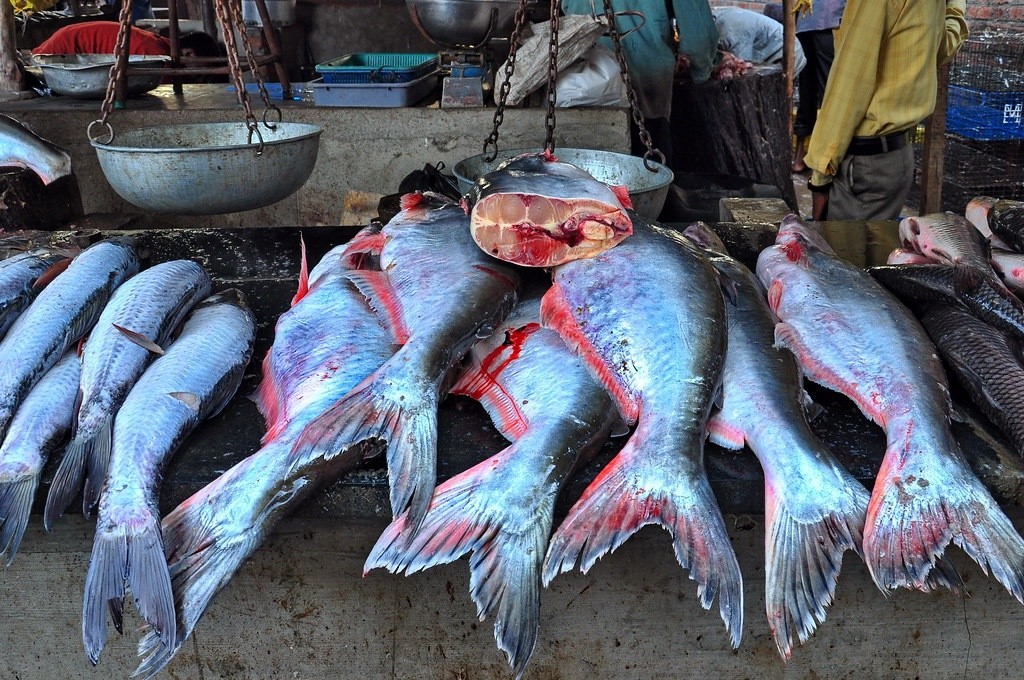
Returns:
[1,111,1024,680]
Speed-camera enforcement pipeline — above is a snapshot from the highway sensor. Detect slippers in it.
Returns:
[792,164,810,174]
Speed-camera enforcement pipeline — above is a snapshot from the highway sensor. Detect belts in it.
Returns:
[844,130,910,156]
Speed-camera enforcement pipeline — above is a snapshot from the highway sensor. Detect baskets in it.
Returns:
[315,51,440,84]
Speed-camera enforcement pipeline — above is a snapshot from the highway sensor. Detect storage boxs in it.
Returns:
[307,52,446,107]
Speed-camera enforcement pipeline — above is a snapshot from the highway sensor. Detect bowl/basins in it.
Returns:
[29,54,173,99]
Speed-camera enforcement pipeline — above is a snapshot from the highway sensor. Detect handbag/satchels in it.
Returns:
[494,13,631,109]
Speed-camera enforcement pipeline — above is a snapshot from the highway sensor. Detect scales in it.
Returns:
[406,0,535,109]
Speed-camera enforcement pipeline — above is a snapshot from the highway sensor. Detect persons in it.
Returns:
[10,0,57,14]
[710,6,807,80]
[104,0,155,26]
[561,0,720,170]
[806,0,970,220]
[32,22,219,85]
[791,0,847,174]
